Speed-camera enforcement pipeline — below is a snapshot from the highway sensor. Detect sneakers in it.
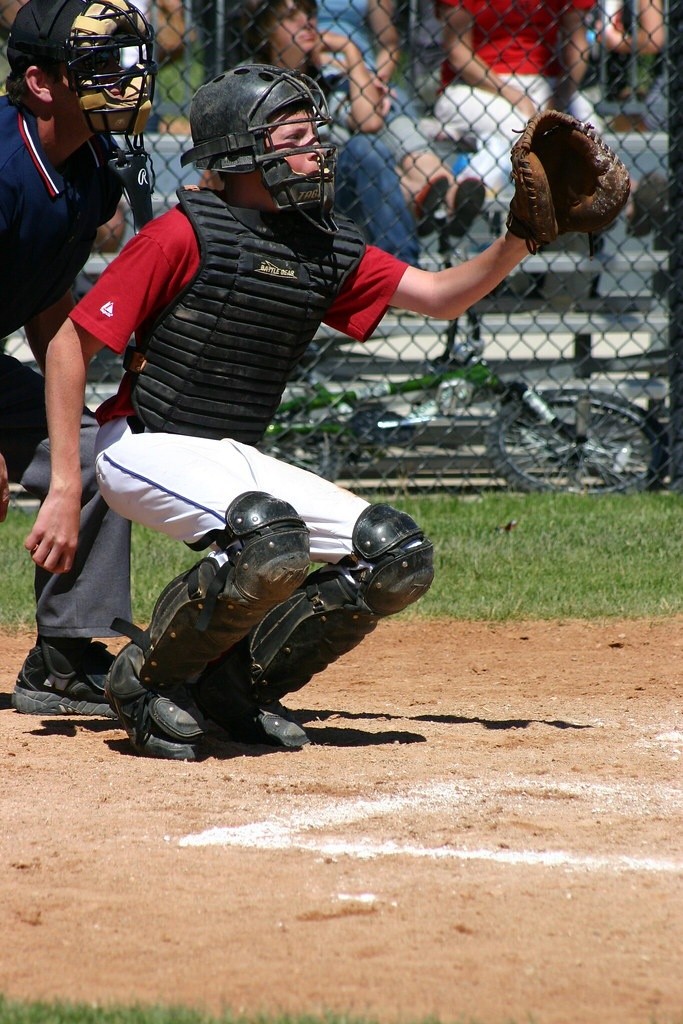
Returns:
[11,641,119,718]
[190,687,307,748]
[105,658,206,762]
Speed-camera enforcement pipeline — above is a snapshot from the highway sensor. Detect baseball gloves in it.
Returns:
[503,109,633,256]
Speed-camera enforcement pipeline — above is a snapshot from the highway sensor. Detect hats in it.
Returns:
[6,0,93,74]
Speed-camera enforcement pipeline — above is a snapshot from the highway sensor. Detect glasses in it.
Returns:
[74,48,120,73]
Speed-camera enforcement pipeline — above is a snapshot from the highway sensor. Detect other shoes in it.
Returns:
[413,176,450,238]
[441,178,485,237]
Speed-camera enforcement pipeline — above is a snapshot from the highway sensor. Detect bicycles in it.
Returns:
[252,259,672,500]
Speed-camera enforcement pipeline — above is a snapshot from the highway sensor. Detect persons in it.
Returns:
[435,0,606,195]
[597,0,666,100]
[21,62,635,760]
[312,0,486,238]
[0,0,160,716]
[253,0,423,266]
[192,0,270,192]
[0,0,202,134]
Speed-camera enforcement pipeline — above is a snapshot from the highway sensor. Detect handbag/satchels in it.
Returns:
[149,0,195,61]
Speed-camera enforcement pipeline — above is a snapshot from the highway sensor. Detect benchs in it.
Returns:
[78,131,667,482]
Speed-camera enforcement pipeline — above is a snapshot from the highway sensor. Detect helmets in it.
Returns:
[180,64,341,237]
